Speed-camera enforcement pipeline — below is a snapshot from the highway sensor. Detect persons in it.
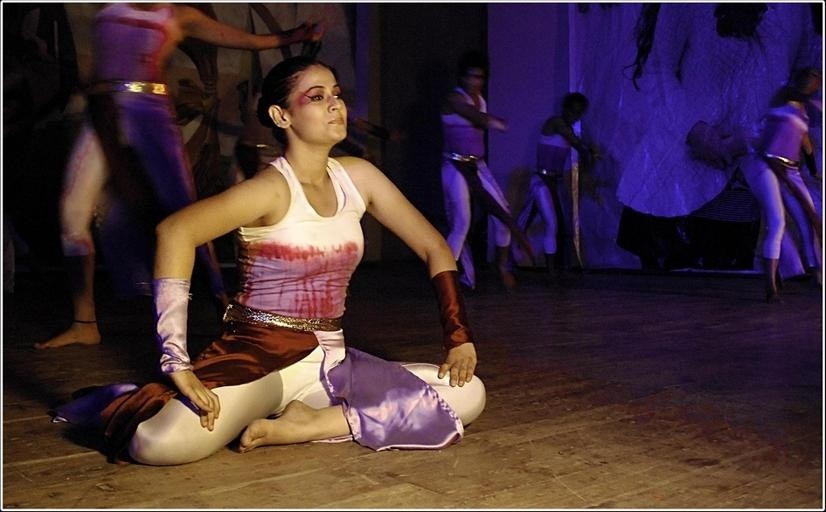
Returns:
[738,68,821,301]
[511,90,599,282]
[431,61,526,290]
[35,6,328,349]
[614,3,826,282]
[51,55,488,466]
